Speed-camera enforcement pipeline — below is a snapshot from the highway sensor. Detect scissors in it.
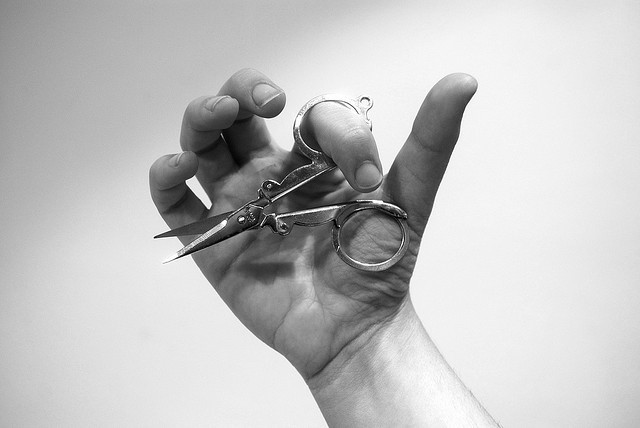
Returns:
[154,95,411,272]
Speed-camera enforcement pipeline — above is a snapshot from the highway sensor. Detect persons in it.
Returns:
[150,66,499,428]
